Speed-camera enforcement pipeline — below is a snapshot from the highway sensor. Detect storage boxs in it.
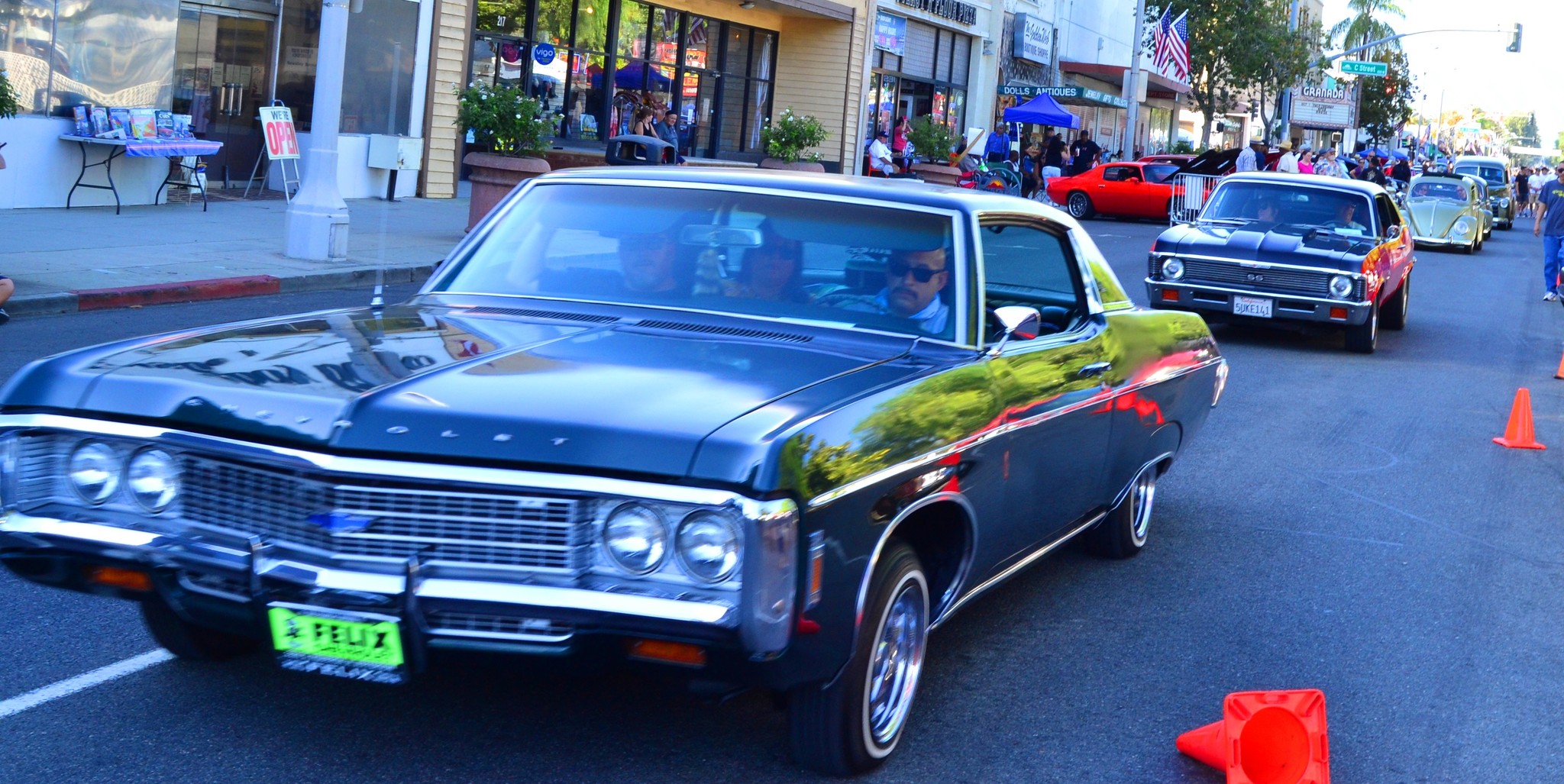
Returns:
[368,134,425,170]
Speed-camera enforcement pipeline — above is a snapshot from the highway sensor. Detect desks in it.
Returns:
[58,134,224,215]
[892,155,928,179]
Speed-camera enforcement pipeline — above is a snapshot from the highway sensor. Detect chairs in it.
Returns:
[949,153,1060,207]
[868,151,888,178]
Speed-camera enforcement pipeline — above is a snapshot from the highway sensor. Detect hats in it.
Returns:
[1135,152,1142,155]
[899,116,910,122]
[1224,140,1229,145]
[1431,161,1437,165]
[1249,136,1336,156]
[663,110,677,120]
[878,131,889,137]
[1081,130,1088,135]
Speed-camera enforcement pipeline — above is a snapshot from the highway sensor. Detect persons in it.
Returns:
[719,216,817,305]
[0,276,14,320]
[628,108,666,164]
[507,215,697,301]
[1534,161,1564,301]
[796,246,949,335]
[868,110,1547,241]
[655,110,688,167]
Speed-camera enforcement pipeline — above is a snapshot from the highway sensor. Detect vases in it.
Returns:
[910,163,962,187]
[761,158,825,173]
[461,152,551,234]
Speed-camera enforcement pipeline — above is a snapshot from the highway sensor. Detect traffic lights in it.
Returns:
[1508,21,1523,51]
[1251,99,1259,121]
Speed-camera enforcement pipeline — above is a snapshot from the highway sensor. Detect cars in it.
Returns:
[1046,148,1282,223]
[1145,171,1416,352]
[1,165,1236,780]
[1398,154,1517,254]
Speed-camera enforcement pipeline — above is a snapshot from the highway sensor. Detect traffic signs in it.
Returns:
[1340,59,1389,77]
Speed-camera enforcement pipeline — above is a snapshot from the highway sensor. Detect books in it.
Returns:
[73,105,195,142]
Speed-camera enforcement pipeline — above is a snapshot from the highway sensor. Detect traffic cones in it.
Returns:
[1553,353,1564,379]
[1492,388,1545,450]
[1175,689,1330,783]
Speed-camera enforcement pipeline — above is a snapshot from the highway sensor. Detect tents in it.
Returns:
[501,59,675,137]
[999,92,1079,186]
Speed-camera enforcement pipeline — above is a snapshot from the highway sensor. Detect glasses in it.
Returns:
[1359,162,1365,164]
[1456,189,1464,193]
[1420,187,1428,191]
[1342,200,1357,208]
[1257,204,1269,210]
[1558,168,1564,173]
[888,257,946,283]
[1369,155,1375,158]
[618,236,672,251]
[1042,156,1046,159]
[759,242,799,261]
[999,127,1003,129]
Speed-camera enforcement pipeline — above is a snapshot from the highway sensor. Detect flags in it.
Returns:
[1153,7,1171,77]
[1168,12,1191,83]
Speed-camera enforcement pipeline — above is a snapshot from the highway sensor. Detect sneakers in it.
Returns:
[1543,291,1556,302]
[0,308,10,321]
[1049,202,1058,207]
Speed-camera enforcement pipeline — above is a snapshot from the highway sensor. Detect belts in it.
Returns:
[989,153,998,155]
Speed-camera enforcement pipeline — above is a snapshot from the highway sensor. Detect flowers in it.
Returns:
[450,79,566,158]
[760,104,833,164]
[906,114,965,164]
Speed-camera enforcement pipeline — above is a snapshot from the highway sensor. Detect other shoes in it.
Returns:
[1516,210,1547,219]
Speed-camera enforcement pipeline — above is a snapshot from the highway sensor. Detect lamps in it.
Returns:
[738,0,755,10]
